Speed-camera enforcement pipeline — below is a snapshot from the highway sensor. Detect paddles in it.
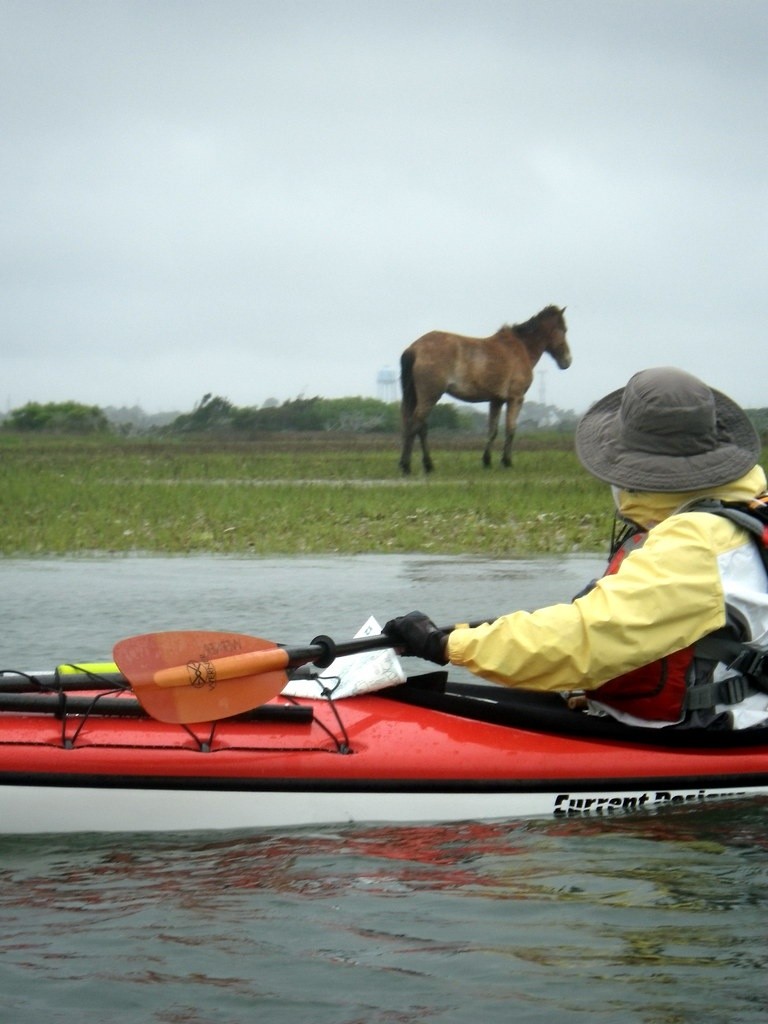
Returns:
[112,617,516,726]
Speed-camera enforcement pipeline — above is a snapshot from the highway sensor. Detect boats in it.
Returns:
[1,647,767,833]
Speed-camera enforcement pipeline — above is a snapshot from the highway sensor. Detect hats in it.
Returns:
[574,366,760,492]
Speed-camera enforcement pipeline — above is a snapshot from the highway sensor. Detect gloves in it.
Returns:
[380,610,450,668]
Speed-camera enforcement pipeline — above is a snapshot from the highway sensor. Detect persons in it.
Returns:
[380,365,768,733]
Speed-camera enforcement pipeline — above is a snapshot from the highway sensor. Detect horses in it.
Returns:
[399,304,573,472]
